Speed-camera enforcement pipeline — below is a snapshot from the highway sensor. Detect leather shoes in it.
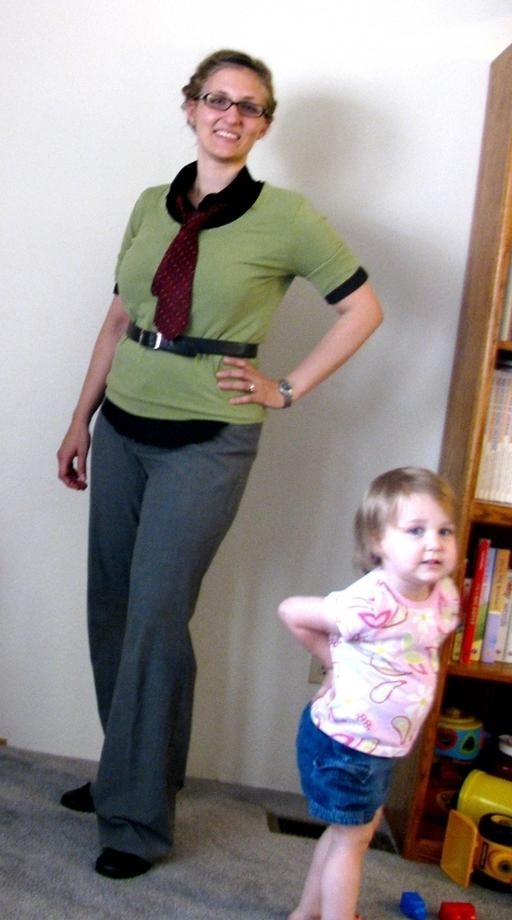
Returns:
[95,845,162,879]
[60,782,97,813]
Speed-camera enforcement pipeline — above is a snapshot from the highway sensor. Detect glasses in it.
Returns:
[194,92,269,119]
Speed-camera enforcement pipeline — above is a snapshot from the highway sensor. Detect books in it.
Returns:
[450,286,512,662]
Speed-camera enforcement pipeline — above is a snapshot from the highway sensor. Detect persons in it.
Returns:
[278,466,462,919]
[55,50,383,878]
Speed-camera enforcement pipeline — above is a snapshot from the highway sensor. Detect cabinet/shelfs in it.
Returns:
[385,44,511,863]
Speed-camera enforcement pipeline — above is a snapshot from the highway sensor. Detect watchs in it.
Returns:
[277,377,294,409]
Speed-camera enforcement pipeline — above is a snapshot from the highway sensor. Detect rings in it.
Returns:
[247,382,255,393]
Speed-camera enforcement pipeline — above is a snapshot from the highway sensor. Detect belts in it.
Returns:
[125,323,259,359]
